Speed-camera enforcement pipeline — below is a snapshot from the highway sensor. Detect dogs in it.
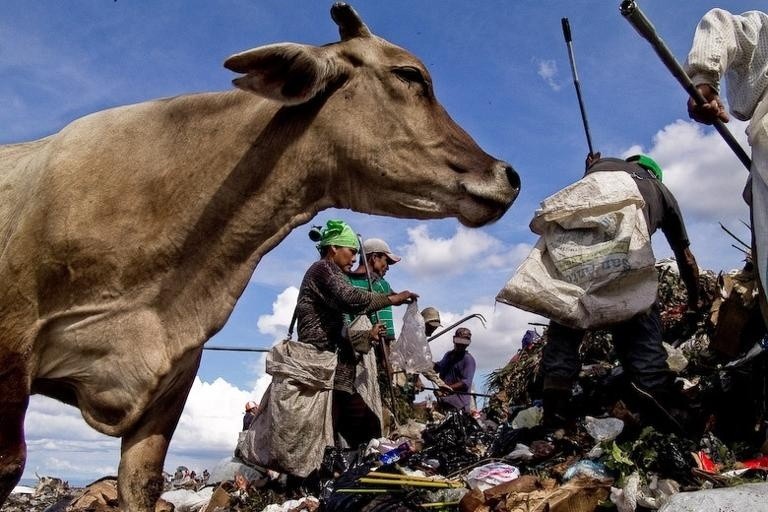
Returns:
[35,471,69,494]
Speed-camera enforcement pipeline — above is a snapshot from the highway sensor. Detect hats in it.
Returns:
[359,239,401,265]
[246,401,258,411]
[626,155,662,182]
[428,321,443,327]
[453,328,471,345]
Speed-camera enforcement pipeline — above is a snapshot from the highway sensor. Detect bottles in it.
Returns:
[369,437,398,456]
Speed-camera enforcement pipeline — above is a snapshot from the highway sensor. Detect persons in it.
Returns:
[293,220,420,445]
[174,469,210,480]
[508,328,542,363]
[422,328,477,412]
[243,400,259,432]
[392,306,455,401]
[538,151,711,426]
[683,7,768,297]
[342,236,403,380]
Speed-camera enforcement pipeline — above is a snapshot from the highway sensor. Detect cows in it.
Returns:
[0,1,522,512]
